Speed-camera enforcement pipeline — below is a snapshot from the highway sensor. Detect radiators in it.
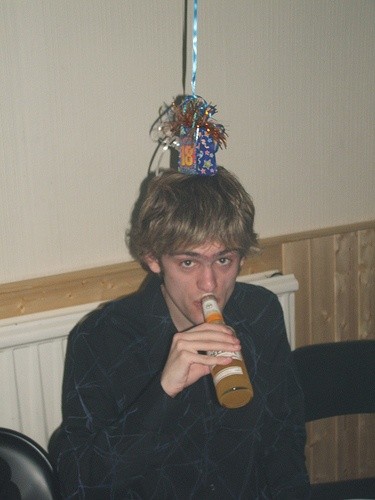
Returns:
[0,269,299,453]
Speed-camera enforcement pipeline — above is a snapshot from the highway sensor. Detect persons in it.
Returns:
[54,165,311,500]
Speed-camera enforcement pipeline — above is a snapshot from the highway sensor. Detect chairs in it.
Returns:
[291,338,374,500]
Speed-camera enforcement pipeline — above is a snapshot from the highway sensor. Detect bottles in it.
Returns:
[200,295,254,409]
